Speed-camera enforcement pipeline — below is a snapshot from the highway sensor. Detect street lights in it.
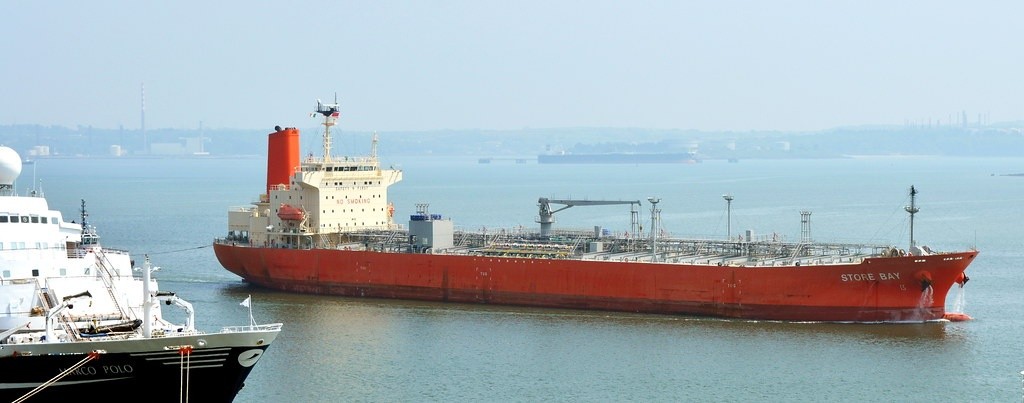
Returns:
[722,195,735,240]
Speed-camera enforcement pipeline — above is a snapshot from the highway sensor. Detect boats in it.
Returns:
[275,202,305,220]
[0,143,284,403]
[942,311,974,322]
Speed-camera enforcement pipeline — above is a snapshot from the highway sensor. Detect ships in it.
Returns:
[210,88,981,323]
